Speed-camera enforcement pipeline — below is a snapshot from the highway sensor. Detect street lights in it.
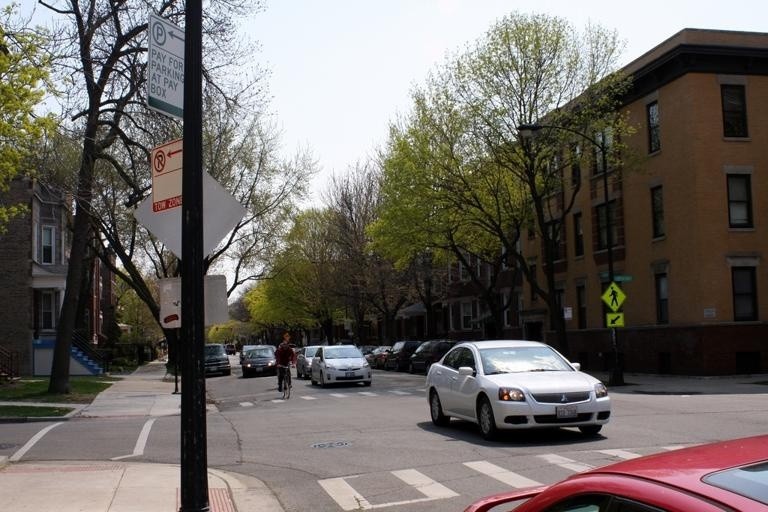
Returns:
[516,122,625,388]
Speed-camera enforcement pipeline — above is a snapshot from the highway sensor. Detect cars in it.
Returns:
[467,430,768,512]
[423,339,612,439]
[205,337,465,388]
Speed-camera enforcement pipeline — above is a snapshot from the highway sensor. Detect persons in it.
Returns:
[273,341,296,392]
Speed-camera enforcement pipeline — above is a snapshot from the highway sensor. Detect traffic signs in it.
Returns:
[146,12,187,121]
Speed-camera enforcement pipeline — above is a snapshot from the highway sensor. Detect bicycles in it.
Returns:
[273,363,296,398]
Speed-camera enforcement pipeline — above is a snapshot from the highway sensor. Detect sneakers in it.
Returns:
[278,385,282,392]
